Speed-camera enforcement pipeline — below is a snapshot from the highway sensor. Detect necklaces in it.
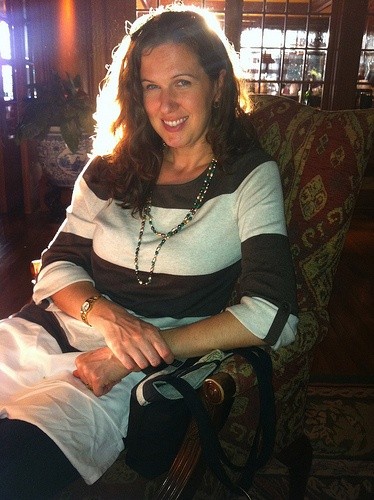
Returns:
[134,152,217,287]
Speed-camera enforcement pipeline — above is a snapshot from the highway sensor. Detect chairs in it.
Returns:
[152,91,374,500]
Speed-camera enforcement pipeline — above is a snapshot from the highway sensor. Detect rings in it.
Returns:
[86,384,93,390]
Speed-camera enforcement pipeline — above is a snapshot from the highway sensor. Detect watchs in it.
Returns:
[80,295,103,326]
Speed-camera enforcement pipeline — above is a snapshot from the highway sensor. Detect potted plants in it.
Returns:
[13,68,93,211]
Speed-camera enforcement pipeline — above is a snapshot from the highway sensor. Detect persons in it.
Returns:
[0,6,300,500]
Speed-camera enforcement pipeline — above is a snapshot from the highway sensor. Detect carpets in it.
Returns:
[53,369,374,500]
